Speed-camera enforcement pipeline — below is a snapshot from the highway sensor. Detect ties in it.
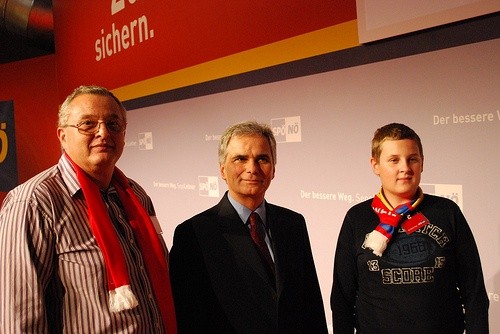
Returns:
[250,213,265,248]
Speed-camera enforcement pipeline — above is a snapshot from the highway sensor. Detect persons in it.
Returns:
[0,86,168,334]
[330,122,490,334]
[168,121,329,334]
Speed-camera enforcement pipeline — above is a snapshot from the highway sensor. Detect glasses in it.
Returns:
[63,118,127,136]
